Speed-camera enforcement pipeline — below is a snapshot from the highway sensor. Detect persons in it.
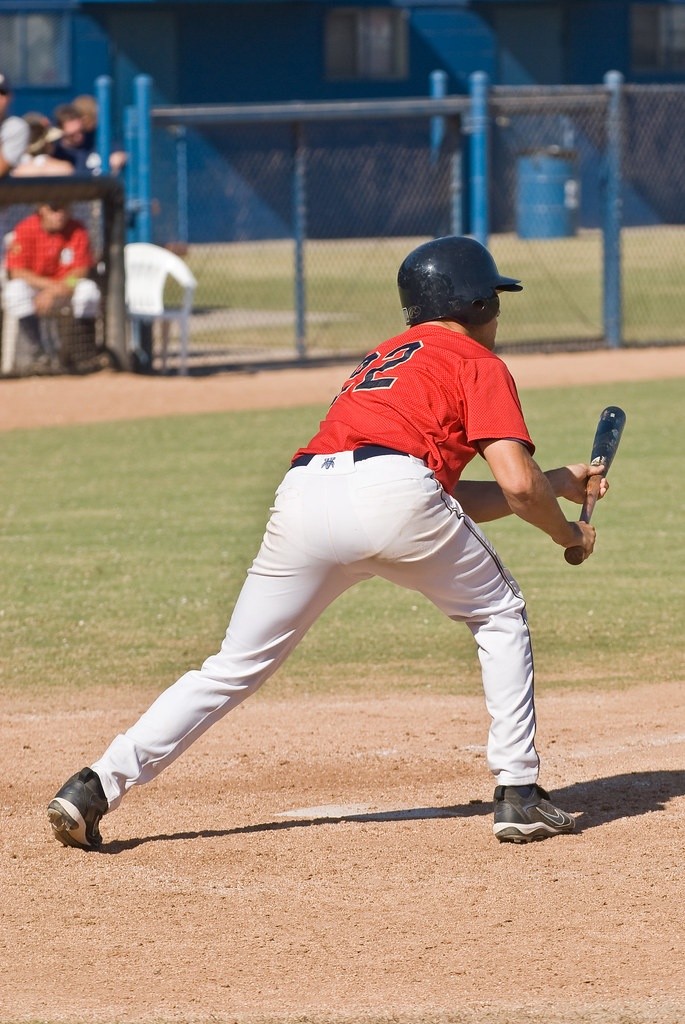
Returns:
[0,71,126,382]
[48,235,611,850]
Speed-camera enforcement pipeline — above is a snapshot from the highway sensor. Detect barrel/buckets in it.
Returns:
[514,145,582,242]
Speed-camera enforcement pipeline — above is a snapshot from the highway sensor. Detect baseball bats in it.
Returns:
[563,405,627,565]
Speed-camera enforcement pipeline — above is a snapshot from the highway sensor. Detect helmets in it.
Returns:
[396,238,522,324]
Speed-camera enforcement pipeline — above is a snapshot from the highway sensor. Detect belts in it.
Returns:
[287,445,402,465]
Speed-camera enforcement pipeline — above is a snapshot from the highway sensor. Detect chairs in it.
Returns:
[125,241,201,380]
[0,231,34,376]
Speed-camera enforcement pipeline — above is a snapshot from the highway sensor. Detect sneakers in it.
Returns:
[495,783,576,843]
[47,768,108,850]
